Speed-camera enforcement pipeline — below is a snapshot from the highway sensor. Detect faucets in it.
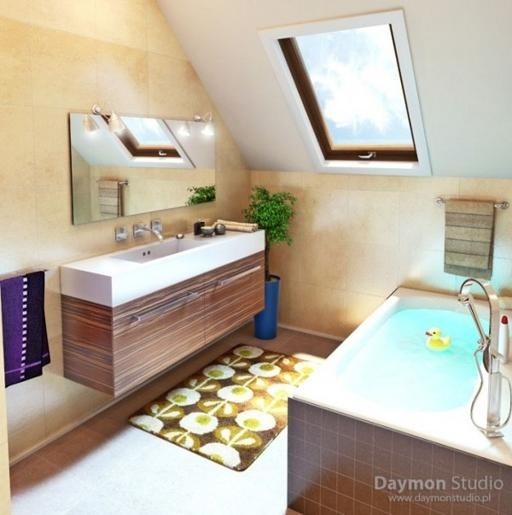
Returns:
[133,222,164,245]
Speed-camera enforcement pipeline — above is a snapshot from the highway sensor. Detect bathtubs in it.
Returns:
[293,286,512,469]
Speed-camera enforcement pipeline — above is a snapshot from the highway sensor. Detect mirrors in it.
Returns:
[69,113,216,225]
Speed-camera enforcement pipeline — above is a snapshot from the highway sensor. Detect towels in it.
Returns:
[443,199,494,281]
[98,179,122,218]
[0,272,51,388]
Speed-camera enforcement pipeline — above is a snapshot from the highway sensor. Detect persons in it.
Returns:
[426,328,452,351]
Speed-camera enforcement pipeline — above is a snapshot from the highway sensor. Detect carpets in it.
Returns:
[126,343,317,471]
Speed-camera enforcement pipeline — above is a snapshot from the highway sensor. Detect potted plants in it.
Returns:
[240,185,296,338]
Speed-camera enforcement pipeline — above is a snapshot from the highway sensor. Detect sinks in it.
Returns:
[109,238,212,264]
